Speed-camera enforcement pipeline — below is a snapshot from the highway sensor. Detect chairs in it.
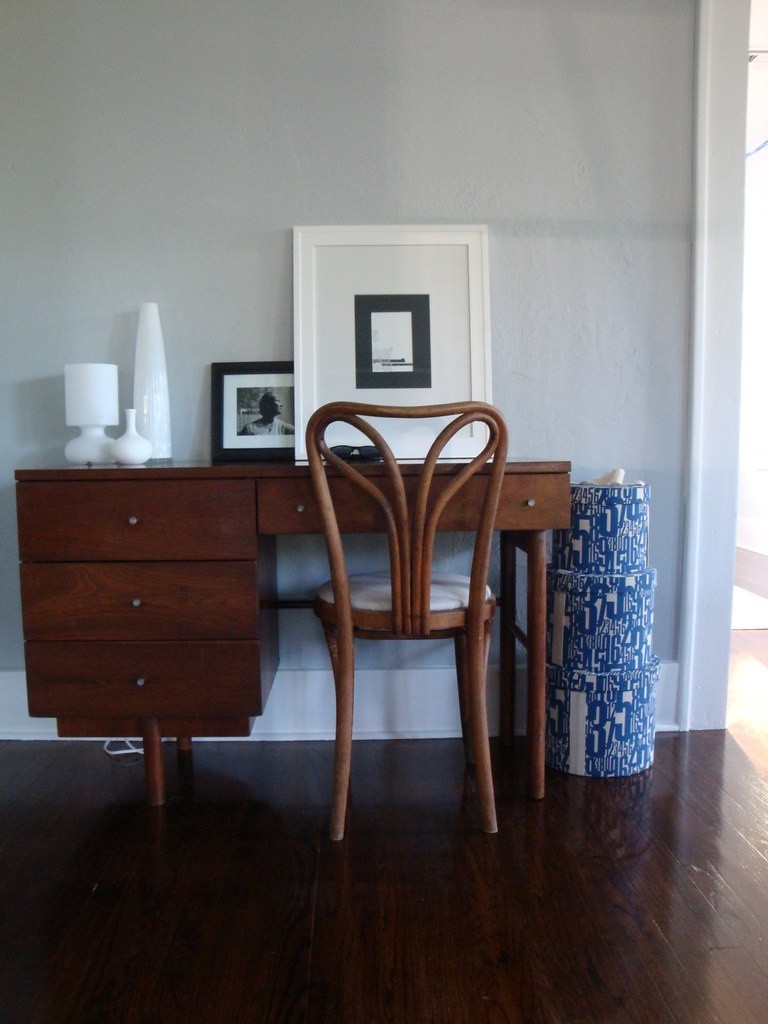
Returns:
[304,402,508,840]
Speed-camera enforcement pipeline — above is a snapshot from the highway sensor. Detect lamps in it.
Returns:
[64,363,119,464]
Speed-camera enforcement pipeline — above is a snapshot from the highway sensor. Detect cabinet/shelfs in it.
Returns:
[14,460,571,805]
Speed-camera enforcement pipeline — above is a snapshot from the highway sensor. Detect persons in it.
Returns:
[238,393,294,434]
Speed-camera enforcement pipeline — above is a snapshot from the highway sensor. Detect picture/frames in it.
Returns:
[210,361,295,463]
[292,224,493,466]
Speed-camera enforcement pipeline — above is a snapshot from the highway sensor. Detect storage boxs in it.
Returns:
[545,469,660,778]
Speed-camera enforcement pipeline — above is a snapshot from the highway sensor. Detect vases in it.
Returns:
[111,409,152,464]
[132,300,172,458]
